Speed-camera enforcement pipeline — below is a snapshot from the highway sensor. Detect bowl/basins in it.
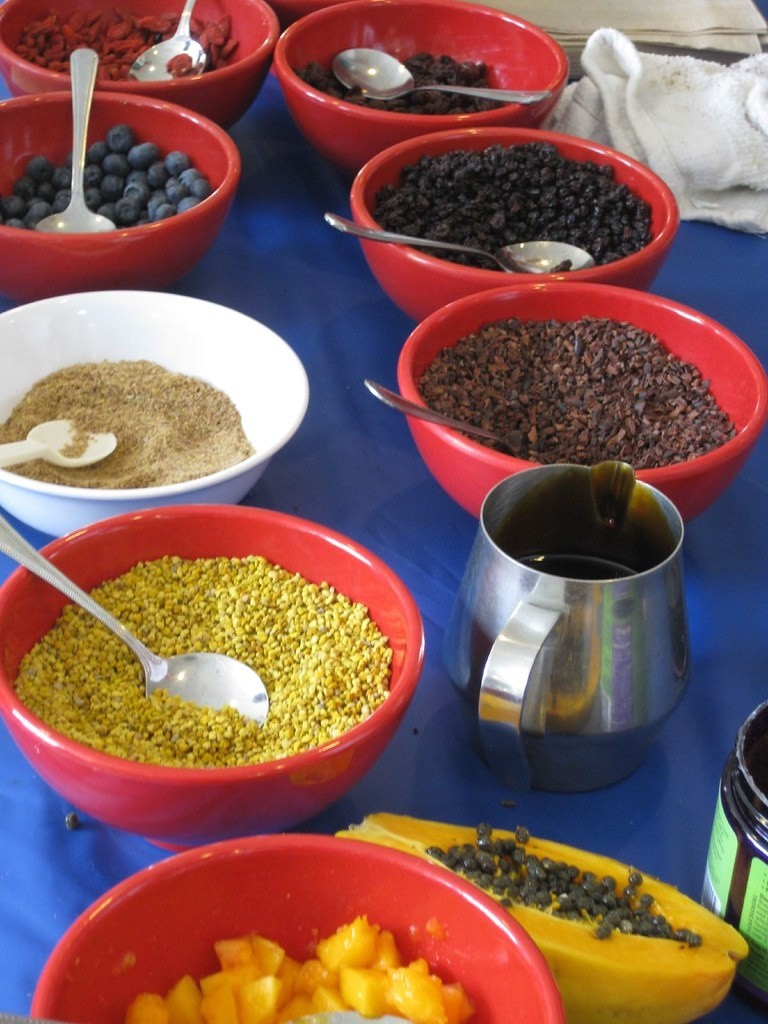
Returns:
[397,283,768,520]
[1,506,427,853]
[272,0,571,173]
[0,91,242,306]
[0,290,310,538]
[30,834,565,1024]
[0,0,281,132]
[349,128,681,325]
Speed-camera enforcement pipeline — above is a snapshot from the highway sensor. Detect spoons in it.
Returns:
[0,509,270,731]
[333,48,552,104]
[0,422,117,468]
[128,1,206,82]
[35,49,116,234]
[324,212,596,273]
[365,381,527,456]
[0,1010,415,1023]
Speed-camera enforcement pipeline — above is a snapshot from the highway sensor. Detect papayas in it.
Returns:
[340,813,748,1024]
[122,918,478,1024]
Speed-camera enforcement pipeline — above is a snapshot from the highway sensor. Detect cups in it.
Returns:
[441,460,692,793]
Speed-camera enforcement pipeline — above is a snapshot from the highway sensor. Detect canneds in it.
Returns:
[696,695,768,1017]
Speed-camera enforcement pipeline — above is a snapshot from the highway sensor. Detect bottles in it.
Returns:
[698,697,768,1011]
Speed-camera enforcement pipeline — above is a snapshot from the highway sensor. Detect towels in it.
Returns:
[544,24,768,239]
